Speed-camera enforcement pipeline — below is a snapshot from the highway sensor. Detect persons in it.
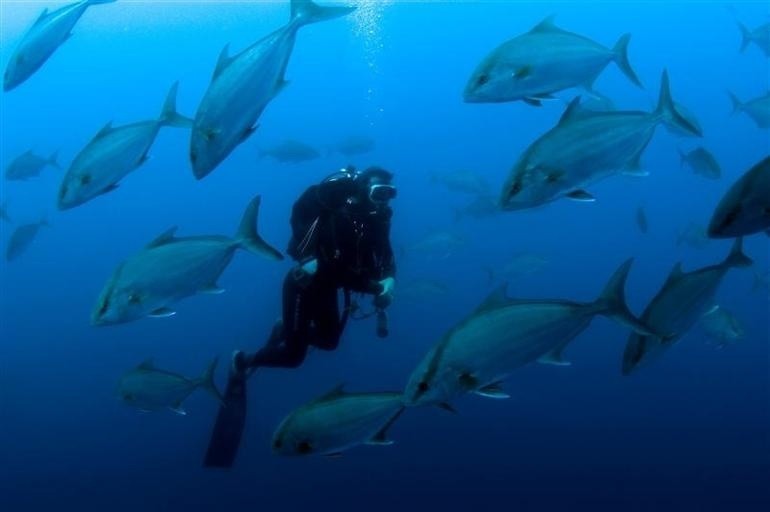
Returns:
[237,166,398,374]
